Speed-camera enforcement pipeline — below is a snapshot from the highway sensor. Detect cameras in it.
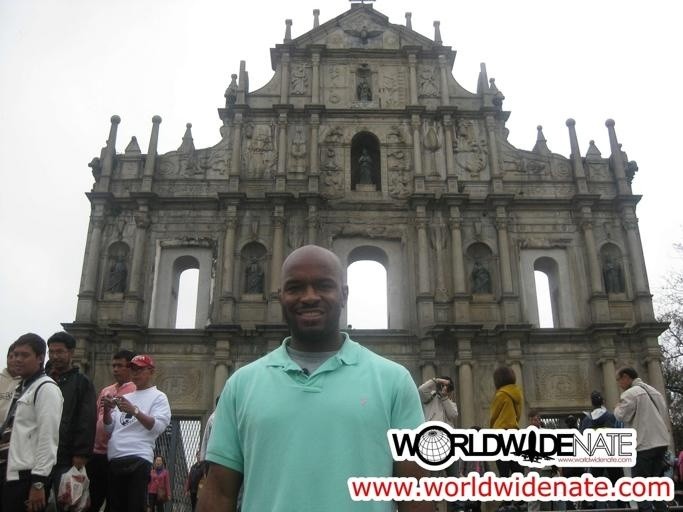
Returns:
[113,397,122,405]
[441,383,450,389]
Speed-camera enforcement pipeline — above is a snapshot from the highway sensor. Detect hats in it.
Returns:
[126,354,156,368]
[591,390,605,403]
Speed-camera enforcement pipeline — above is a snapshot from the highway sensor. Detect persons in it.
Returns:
[417,376,459,512]
[612,367,672,512]
[604,250,622,294]
[487,366,523,512]
[524,410,547,512]
[358,154,373,183]
[106,248,125,292]
[579,391,622,511]
[469,260,491,295]
[458,425,487,512]
[193,243,434,512]
[243,253,263,294]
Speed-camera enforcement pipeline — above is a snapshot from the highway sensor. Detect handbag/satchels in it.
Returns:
[157,487,168,503]
[58,471,85,505]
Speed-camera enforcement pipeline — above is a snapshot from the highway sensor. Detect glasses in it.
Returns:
[46,350,69,353]
